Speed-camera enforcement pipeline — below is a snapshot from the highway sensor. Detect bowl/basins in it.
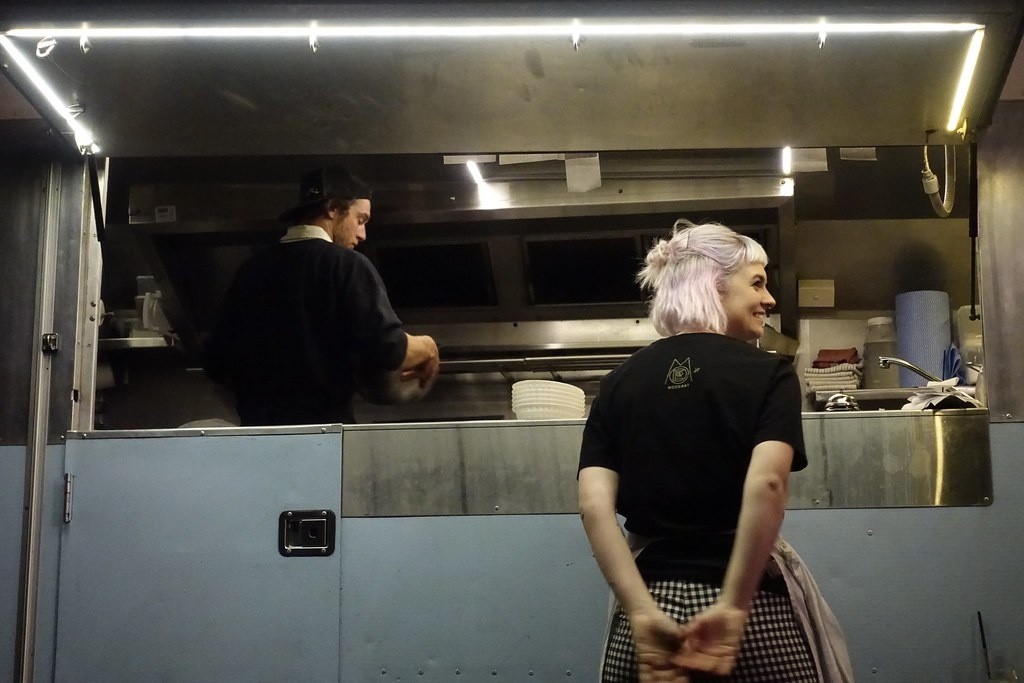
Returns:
[356,363,431,406]
[134,275,158,328]
[511,380,585,421]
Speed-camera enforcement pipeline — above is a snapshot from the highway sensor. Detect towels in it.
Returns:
[803,346,863,391]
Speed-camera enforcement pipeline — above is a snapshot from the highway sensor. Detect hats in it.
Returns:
[280,166,351,221]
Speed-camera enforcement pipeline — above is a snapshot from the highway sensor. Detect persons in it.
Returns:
[575,220,854,683]
[204,165,439,428]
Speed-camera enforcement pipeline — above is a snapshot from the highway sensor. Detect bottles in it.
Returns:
[862,316,900,388]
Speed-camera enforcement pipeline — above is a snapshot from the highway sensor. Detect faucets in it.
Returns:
[878,356,957,392]
